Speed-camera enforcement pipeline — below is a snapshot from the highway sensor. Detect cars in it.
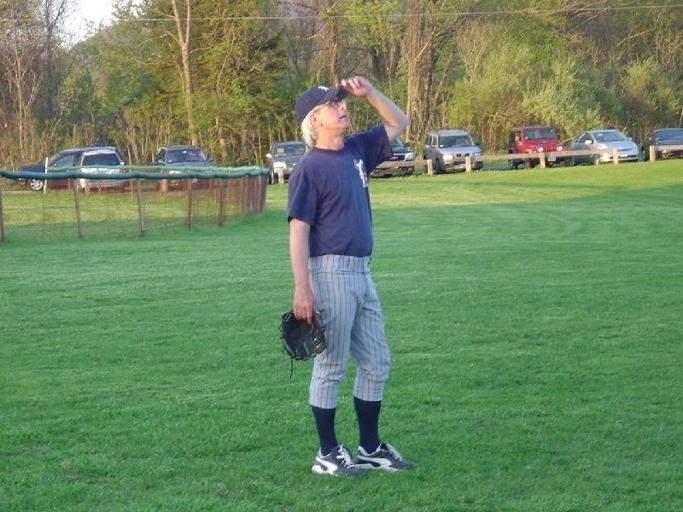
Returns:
[151,145,222,192]
[261,140,308,185]
[641,128,683,163]
[559,129,639,167]
[70,149,124,196]
[17,146,128,194]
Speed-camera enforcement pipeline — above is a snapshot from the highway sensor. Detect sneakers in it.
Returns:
[311,443,360,478]
[352,442,417,471]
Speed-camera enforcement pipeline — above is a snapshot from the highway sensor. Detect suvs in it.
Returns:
[504,125,567,173]
[369,137,415,179]
[422,128,483,174]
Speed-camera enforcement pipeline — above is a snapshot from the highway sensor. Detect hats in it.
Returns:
[294,84,350,129]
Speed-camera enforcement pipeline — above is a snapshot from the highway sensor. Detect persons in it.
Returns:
[287,75,417,477]
